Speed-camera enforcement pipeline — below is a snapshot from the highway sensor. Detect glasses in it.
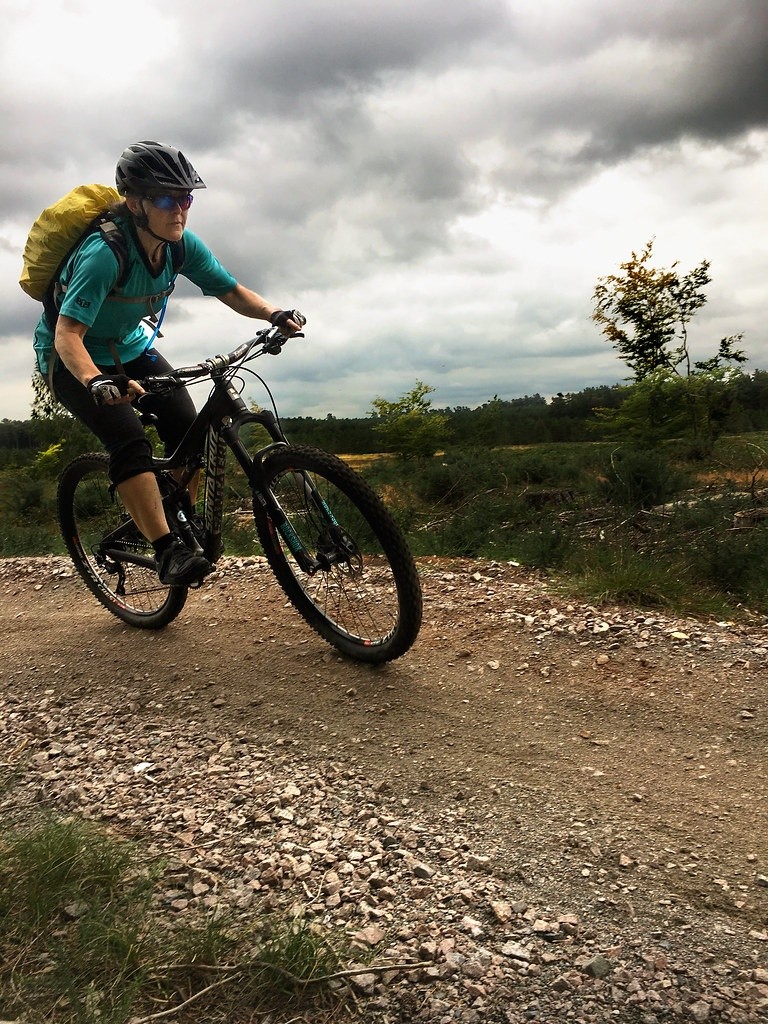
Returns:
[144,193,194,211]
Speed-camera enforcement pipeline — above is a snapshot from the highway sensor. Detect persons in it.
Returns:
[32,140,307,587]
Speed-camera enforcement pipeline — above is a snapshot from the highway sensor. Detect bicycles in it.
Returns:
[55,329,423,666]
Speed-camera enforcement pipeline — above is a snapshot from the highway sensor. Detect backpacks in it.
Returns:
[18,183,186,403]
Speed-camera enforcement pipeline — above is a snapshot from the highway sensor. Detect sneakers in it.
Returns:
[154,536,210,585]
[219,539,225,553]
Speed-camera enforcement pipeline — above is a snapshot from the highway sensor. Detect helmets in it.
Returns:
[115,140,207,200]
